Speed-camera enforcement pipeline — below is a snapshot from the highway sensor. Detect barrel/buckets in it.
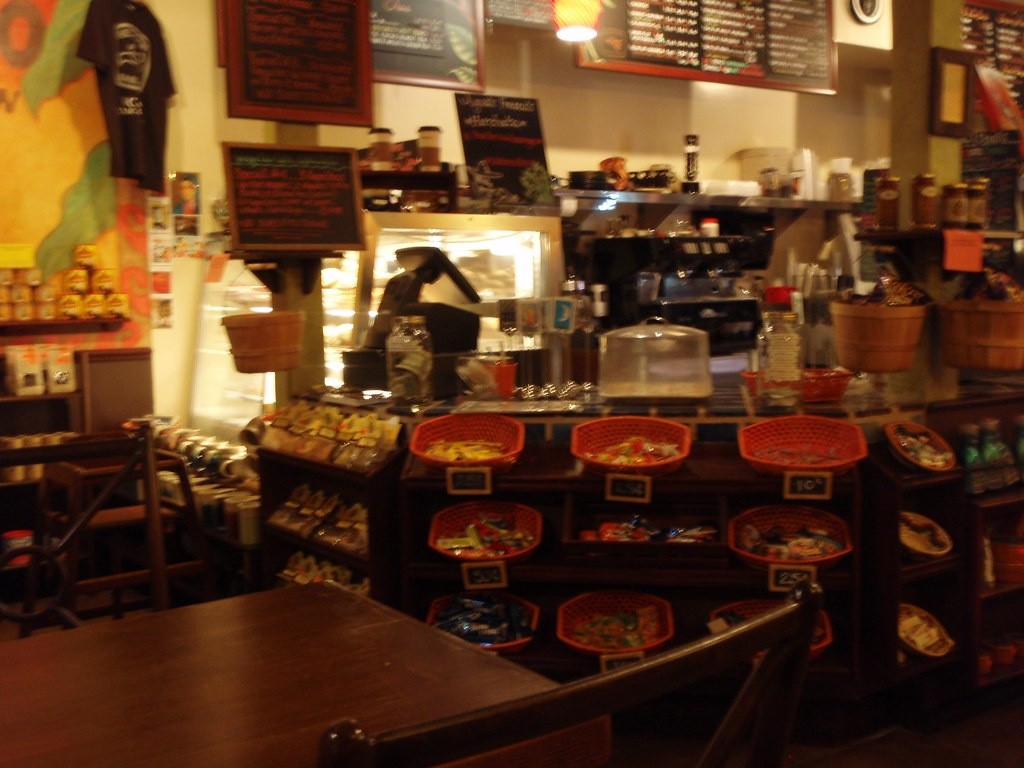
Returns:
[221,311,306,373]
[826,298,932,372]
[940,296,1023,371]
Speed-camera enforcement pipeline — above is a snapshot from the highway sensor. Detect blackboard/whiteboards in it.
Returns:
[366,1,487,93]
[225,0,375,126]
[221,140,369,251]
[580,0,841,95]
[455,93,548,198]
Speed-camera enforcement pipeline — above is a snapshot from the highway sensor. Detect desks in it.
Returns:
[0,581,604,768]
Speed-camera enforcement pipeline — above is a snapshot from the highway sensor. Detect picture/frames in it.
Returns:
[928,48,979,139]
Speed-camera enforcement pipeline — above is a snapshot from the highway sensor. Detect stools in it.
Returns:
[15,445,226,642]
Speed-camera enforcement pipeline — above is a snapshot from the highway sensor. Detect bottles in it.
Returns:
[751,313,802,412]
[560,279,593,334]
[700,217,720,239]
[385,318,433,407]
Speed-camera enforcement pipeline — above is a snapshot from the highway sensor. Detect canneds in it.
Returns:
[0,431,79,485]
[873,172,990,231]
[0,243,131,323]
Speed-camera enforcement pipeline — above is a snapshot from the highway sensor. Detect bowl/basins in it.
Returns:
[886,419,956,476]
[900,511,953,558]
[897,603,953,659]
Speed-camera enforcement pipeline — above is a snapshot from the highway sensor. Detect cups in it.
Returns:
[419,126,441,172]
[367,128,394,172]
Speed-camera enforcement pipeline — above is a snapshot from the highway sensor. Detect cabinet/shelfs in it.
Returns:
[396,442,864,748]
[973,483,1024,715]
[252,397,407,612]
[870,449,987,738]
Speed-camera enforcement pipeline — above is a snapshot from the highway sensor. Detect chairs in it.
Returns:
[316,580,823,768]
